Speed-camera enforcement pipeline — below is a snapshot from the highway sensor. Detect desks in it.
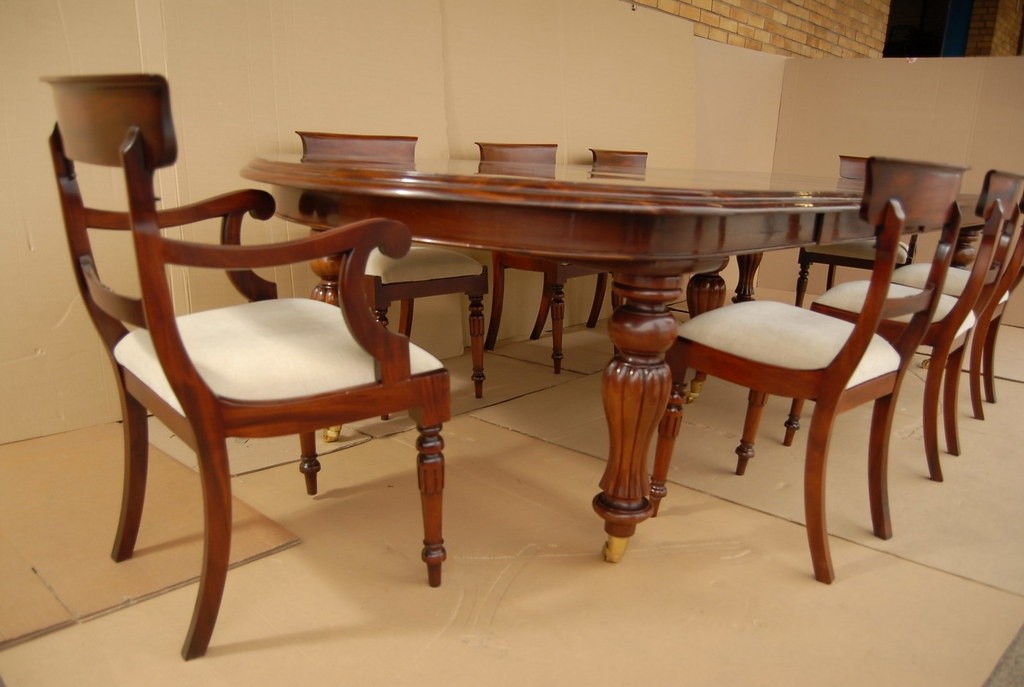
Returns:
[243,153,987,563]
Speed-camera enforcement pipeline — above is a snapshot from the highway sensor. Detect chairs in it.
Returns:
[477,141,622,374]
[585,149,649,328]
[888,198,1024,420]
[795,155,920,307]
[40,74,451,663]
[781,169,1024,482]
[648,155,968,586]
[295,130,490,420]
